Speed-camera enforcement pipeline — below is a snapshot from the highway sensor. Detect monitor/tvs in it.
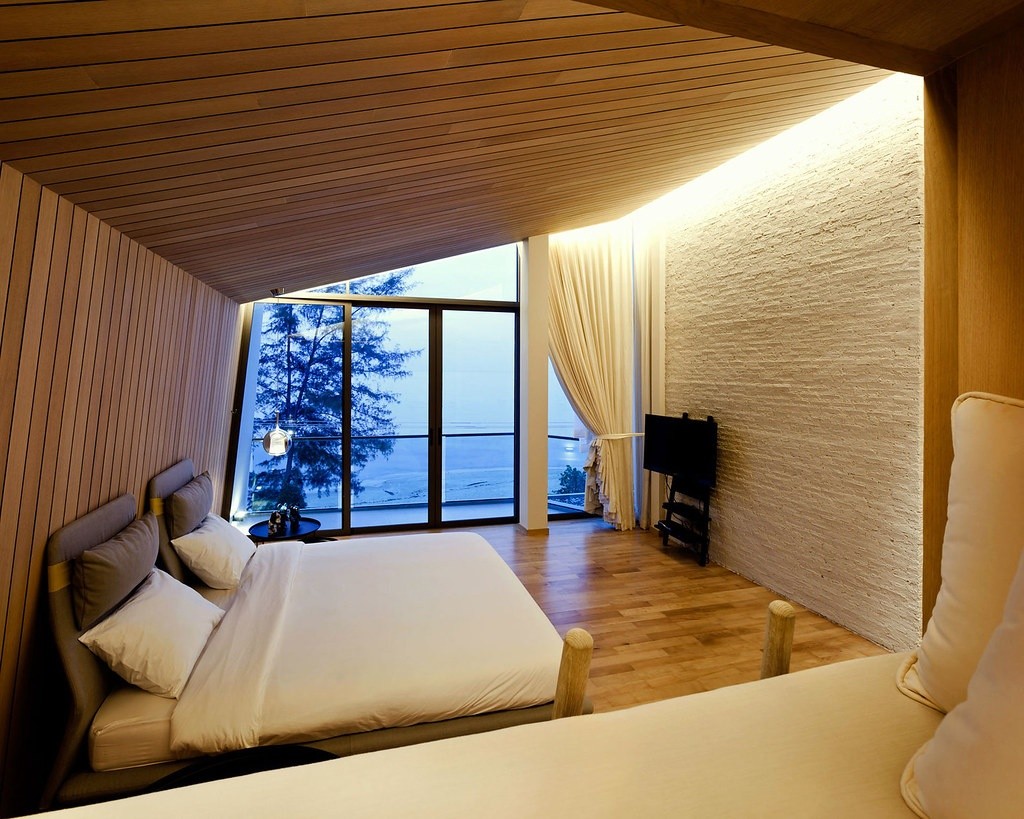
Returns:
[644,414,718,487]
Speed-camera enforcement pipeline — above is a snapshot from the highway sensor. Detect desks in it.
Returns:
[251,530,318,548]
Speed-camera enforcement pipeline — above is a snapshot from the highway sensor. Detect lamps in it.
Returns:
[262,286,293,457]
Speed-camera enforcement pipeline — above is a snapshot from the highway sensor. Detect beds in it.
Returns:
[29,457,595,815]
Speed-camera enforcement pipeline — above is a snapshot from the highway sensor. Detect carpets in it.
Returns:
[254,535,341,548]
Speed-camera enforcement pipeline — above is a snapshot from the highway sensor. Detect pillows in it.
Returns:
[896,390,1024,715]
[69,510,159,631]
[167,470,214,540]
[169,510,256,591]
[78,565,228,700]
[899,538,1024,818]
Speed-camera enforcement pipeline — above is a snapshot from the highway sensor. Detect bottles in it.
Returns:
[279,505,287,525]
[290,506,299,531]
